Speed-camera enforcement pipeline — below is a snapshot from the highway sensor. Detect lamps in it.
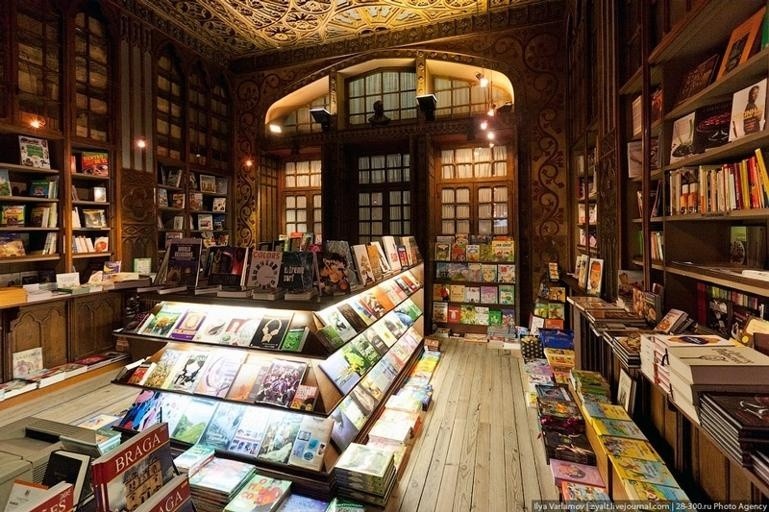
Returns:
[309,108,332,131]
[416,93,438,120]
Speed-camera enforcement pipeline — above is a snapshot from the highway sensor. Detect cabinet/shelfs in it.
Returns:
[619,0,768,321]
[570,119,599,259]
[112,260,426,502]
[154,154,234,274]
[433,234,518,334]
[0,122,122,384]
